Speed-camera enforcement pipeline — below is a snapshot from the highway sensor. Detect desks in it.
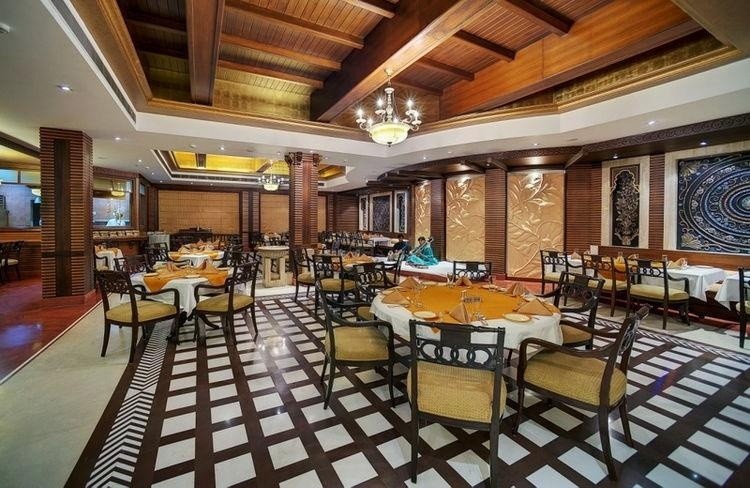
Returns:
[721,272,750,320]
[630,261,725,318]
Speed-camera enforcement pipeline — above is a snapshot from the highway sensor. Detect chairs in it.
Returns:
[193,260,259,345]
[292,245,319,303]
[0,241,10,284]
[89,225,291,336]
[513,306,650,481]
[406,319,507,485]
[95,270,180,362]
[3,240,25,282]
[370,258,564,400]
[303,228,413,315]
[736,267,750,348]
[554,270,605,350]
[316,281,396,409]
[625,257,691,330]
[539,248,626,316]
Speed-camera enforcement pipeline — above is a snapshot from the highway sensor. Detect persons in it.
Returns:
[106,211,126,226]
[392,234,409,255]
[414,236,432,259]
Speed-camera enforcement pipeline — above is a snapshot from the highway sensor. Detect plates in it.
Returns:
[411,308,439,319]
[502,311,532,322]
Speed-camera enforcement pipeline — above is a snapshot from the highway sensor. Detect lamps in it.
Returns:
[355,66,422,149]
[257,159,285,193]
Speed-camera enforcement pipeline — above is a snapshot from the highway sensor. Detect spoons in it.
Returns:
[478,313,489,326]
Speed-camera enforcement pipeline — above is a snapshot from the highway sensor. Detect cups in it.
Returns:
[618,251,623,256]
[446,270,454,288]
[488,274,496,292]
[680,257,688,269]
